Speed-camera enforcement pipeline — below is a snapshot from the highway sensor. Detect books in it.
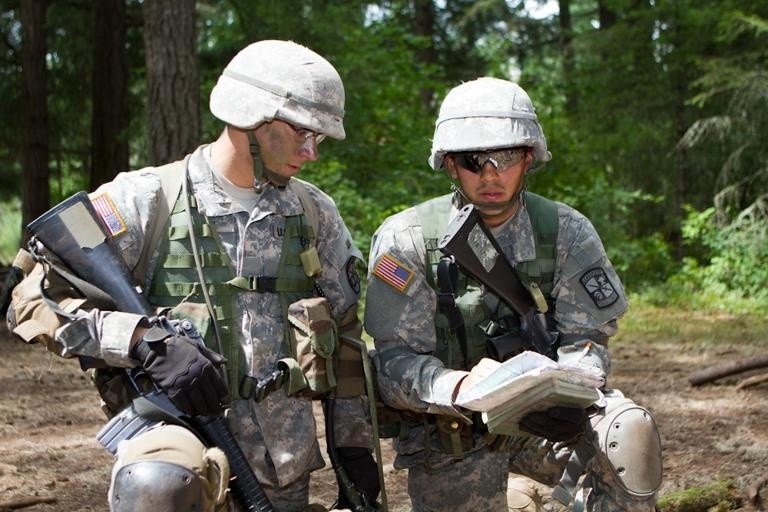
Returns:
[451,351,606,439]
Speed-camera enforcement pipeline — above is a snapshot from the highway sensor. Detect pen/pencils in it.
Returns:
[578,342,592,361]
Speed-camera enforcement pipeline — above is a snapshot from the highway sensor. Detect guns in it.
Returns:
[438,201,558,362]
[27,190,274,510]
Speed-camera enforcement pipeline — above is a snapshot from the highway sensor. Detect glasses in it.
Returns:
[451,149,526,174]
[287,125,327,143]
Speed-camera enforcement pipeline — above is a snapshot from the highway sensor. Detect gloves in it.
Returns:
[333,444,383,511]
[131,323,229,413]
[519,404,586,443]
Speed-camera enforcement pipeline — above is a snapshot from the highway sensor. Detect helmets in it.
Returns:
[428,76,554,167]
[210,40,347,142]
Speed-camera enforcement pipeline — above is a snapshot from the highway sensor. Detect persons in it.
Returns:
[363,76,662,512]
[5,39,380,512]
